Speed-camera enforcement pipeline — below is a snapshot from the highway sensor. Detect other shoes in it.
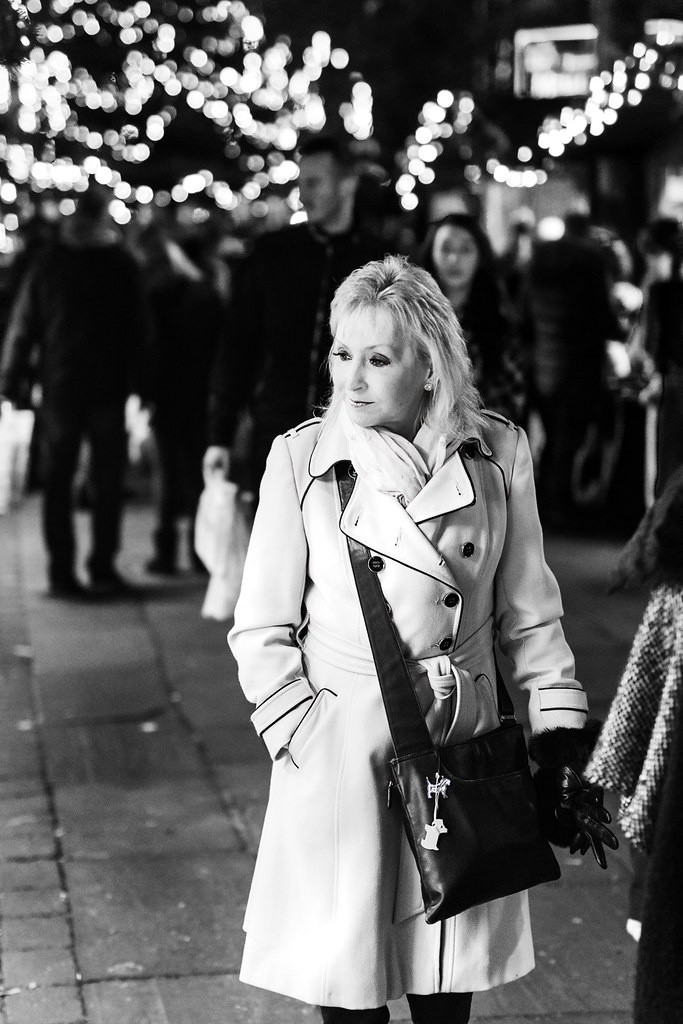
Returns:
[47,566,97,601]
[87,560,144,600]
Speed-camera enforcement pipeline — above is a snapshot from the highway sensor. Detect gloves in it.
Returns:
[524,719,619,869]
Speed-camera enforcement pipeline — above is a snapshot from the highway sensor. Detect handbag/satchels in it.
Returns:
[193,465,250,624]
[386,721,562,926]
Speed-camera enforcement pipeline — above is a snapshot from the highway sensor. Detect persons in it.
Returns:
[1,149,683,601]
[223,258,617,1024]
[584,476,681,1024]
